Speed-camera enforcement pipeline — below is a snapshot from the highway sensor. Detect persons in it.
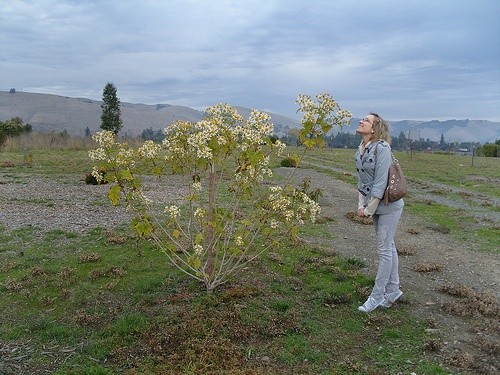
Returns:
[353,113,406,313]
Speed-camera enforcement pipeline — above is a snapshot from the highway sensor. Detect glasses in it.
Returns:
[363,118,373,124]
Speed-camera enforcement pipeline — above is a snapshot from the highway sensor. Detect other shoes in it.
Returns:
[380,290,403,308]
[358,296,385,312]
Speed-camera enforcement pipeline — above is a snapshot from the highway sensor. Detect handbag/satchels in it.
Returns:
[375,142,406,206]
